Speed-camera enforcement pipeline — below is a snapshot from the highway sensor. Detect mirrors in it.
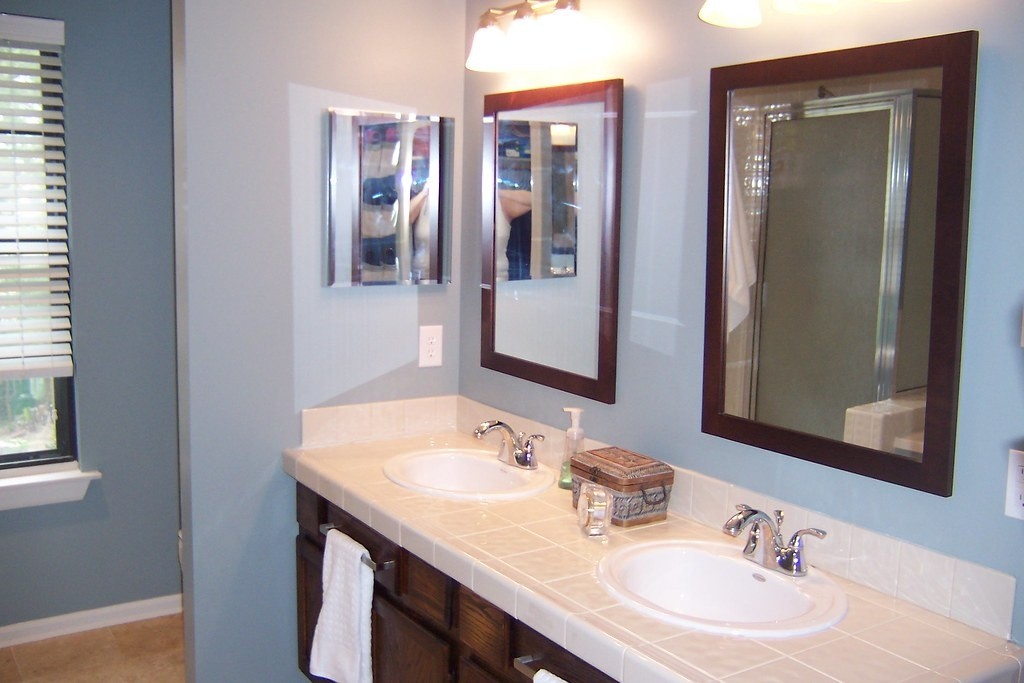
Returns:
[696,30,982,498]
[323,104,457,287]
[348,114,446,286]
[478,77,626,406]
[494,118,579,283]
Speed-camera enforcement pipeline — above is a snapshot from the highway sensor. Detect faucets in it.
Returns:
[720,502,788,574]
[472,419,524,465]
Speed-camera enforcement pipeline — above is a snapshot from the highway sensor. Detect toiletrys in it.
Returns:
[558,405,587,489]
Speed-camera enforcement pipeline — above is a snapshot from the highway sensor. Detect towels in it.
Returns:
[534,668,568,682]
[730,149,757,353]
[308,527,379,683]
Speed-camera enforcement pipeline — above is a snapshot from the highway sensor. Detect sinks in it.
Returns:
[380,446,557,500]
[595,532,851,643]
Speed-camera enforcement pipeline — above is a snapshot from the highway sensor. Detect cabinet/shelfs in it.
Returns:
[292,477,624,683]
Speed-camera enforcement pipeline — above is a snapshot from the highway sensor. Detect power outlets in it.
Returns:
[1003,447,1024,523]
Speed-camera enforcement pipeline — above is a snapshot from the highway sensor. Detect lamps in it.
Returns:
[463,1,597,75]
[696,1,819,31]
